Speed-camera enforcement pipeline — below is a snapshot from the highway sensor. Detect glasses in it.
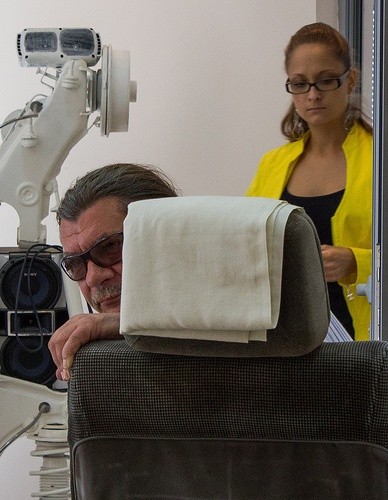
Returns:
[59,232,124,281]
[285,68,349,95]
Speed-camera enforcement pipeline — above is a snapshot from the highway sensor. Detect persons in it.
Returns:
[46,161,355,380]
[243,23,375,341]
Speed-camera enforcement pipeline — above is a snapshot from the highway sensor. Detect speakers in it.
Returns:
[0,247,70,392]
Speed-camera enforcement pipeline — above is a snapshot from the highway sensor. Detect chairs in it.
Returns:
[67,196,388,500]
